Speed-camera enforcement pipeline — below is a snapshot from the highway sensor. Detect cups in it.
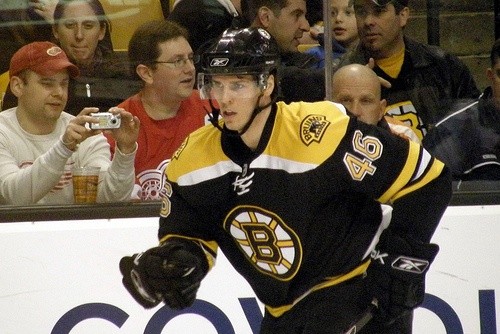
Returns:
[71,166,101,204]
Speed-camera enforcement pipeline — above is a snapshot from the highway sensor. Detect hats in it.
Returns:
[9,42,79,79]
[347,0,390,8]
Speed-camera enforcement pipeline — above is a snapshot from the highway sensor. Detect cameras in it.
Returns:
[88,112,121,131]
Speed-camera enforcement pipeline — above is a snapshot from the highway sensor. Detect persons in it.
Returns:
[160,0,238,86]
[341,1,483,147]
[131,27,454,334]
[0,41,140,209]
[1,0,114,121]
[422,39,500,183]
[0,0,60,76]
[322,62,423,162]
[91,20,223,202]
[301,0,360,74]
[227,0,335,103]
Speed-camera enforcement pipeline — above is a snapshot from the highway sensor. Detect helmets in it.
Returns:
[196,26,282,100]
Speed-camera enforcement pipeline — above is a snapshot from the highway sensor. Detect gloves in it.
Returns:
[119,238,208,310]
[356,230,439,326]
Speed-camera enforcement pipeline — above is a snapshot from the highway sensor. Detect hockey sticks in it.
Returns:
[341,304,375,334]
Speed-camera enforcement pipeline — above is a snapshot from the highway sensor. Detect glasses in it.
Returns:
[142,55,201,68]
